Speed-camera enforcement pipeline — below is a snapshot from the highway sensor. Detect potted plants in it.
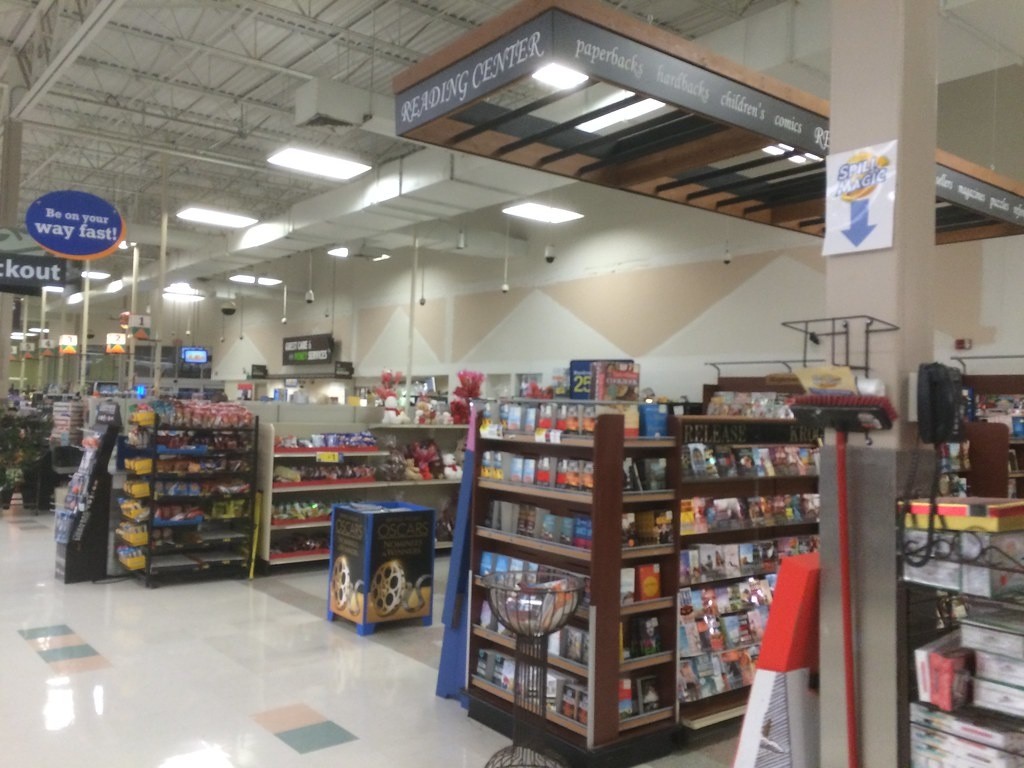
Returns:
[0,412,55,510]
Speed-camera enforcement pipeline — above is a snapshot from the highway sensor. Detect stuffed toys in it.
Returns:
[405,436,467,480]
[436,498,458,542]
[381,396,454,424]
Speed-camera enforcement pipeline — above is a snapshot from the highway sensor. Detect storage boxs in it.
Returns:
[914,608,1024,719]
[51,401,84,445]
[480,450,667,494]
[896,496,1024,597]
[482,400,669,440]
[680,536,819,703]
[909,703,1024,768]
[479,550,661,610]
[472,581,673,734]
[681,494,819,536]
[591,362,641,400]
[483,499,673,553]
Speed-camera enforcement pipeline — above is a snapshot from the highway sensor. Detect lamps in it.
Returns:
[266,145,372,181]
[28,328,49,333]
[327,248,391,261]
[228,274,283,286]
[531,63,589,90]
[81,271,111,279]
[42,286,64,293]
[502,199,585,224]
[9,332,38,340]
[175,208,258,229]
[161,293,204,304]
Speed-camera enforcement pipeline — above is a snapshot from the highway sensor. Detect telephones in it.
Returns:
[917,362,965,445]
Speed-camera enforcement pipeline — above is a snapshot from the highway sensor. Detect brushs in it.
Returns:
[788,393,899,768]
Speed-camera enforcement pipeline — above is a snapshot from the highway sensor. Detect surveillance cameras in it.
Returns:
[86,331,95,339]
[222,301,237,315]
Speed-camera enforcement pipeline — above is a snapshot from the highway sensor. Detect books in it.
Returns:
[571,360,640,402]
[481,599,591,666]
[705,391,797,419]
[483,399,660,438]
[911,609,1023,768]
[479,497,674,548]
[481,451,666,494]
[474,650,579,720]
[479,553,659,608]
[679,441,822,705]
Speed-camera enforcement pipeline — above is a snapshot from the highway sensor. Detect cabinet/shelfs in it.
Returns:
[896,530,1024,768]
[114,414,259,587]
[676,416,823,749]
[936,374,1024,496]
[256,423,469,577]
[460,395,681,768]
